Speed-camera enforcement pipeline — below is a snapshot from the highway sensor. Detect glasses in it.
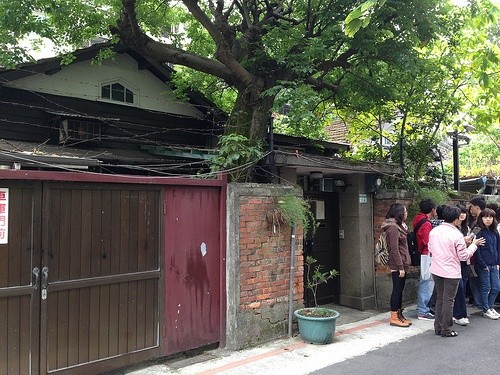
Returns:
[404,210,409,214]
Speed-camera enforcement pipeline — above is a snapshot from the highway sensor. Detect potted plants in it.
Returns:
[293,256,342,345]
[266,188,318,246]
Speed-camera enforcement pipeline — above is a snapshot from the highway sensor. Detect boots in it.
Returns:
[390,308,412,327]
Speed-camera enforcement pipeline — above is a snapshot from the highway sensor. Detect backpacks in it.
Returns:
[374,231,390,268]
[407,218,432,266]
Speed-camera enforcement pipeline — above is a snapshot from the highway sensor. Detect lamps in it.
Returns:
[334,178,351,191]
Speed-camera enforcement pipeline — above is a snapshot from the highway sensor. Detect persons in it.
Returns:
[428,206,486,337]
[412,199,436,321]
[465,197,500,305]
[427,205,448,317]
[452,205,477,326]
[475,208,500,320]
[381,203,412,327]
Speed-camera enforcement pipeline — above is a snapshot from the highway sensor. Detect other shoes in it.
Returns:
[452,317,469,323]
[428,307,436,316]
[454,318,467,325]
[441,330,458,337]
[417,313,435,320]
[482,308,500,319]
[436,331,442,335]
